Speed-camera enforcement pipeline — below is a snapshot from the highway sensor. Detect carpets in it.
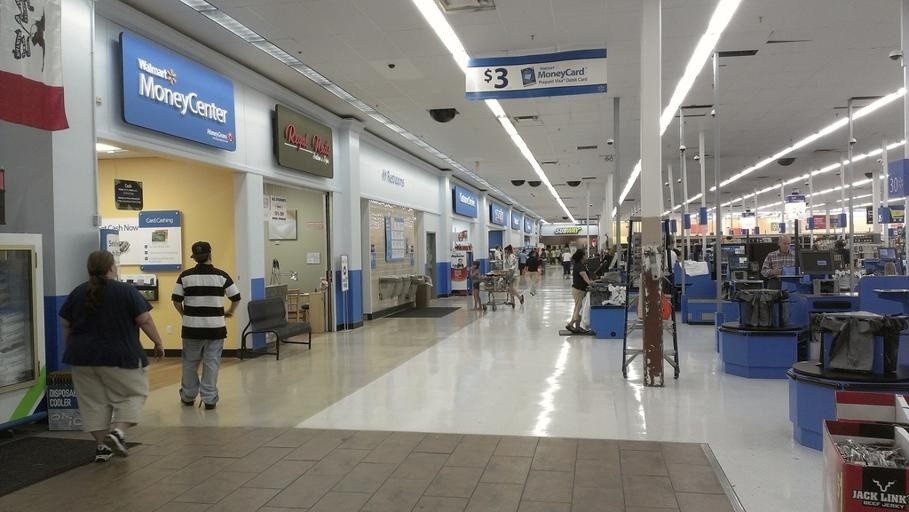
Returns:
[386,309,462,319]
[0,433,142,498]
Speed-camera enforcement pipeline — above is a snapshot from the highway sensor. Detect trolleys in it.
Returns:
[480,272,517,313]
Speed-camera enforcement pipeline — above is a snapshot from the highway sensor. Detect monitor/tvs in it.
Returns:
[679,238,898,274]
[594,260,609,276]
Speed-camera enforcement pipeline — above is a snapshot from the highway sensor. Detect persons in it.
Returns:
[519,247,572,296]
[760,234,795,290]
[494,244,505,270]
[565,249,595,334]
[469,261,482,310]
[457,258,464,268]
[57,251,165,463]
[503,244,524,305]
[170,241,242,410]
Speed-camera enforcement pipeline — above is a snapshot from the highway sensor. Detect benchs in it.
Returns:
[240,298,312,361]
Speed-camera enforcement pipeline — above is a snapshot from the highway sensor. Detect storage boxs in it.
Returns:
[821,418,908,511]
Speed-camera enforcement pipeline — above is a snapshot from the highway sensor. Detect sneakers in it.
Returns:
[177,389,194,405]
[565,324,578,333]
[519,295,524,305]
[94,447,113,462]
[576,326,589,334]
[103,430,128,458]
[204,405,216,409]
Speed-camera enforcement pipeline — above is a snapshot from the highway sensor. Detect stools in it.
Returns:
[588,299,631,339]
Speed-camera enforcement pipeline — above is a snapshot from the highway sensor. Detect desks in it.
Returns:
[713,324,812,382]
[784,359,909,450]
[669,233,909,450]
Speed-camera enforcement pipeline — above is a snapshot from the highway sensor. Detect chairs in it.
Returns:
[286,288,307,323]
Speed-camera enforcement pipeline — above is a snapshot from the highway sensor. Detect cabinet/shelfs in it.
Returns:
[311,289,329,331]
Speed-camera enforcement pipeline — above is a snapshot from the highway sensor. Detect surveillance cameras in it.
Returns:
[889,50,903,62]
[665,107,715,185]
[849,137,856,145]
[607,139,613,145]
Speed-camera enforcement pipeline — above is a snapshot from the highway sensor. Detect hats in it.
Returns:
[190,241,211,258]
